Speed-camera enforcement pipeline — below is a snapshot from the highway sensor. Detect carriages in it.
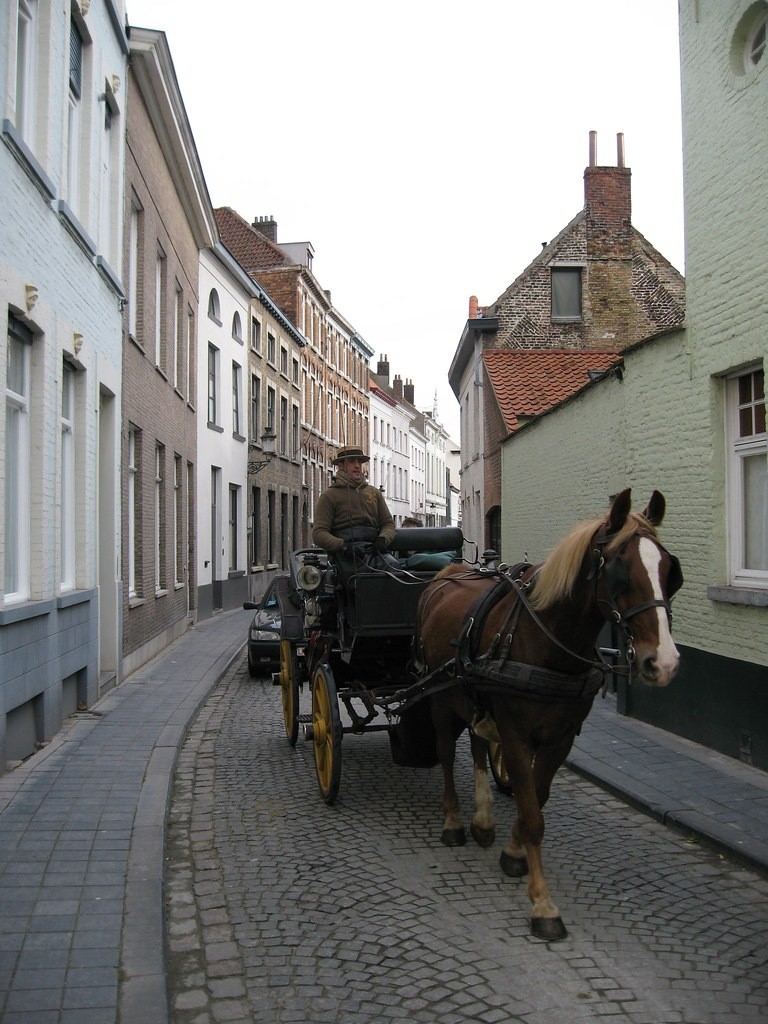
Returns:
[270,486,685,941]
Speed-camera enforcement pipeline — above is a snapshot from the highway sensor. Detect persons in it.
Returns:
[312,445,401,577]
[395,517,423,560]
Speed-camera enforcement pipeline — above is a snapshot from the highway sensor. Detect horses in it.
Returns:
[415,487,684,944]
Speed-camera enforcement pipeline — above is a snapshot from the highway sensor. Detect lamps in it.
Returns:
[379,485,385,499]
[427,503,437,521]
[248,427,277,478]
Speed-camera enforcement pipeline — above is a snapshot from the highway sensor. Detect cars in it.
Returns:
[243,574,306,678]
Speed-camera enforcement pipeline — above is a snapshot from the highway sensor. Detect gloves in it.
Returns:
[343,545,365,561]
[364,537,387,556]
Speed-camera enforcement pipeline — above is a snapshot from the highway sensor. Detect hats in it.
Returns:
[331,446,370,465]
